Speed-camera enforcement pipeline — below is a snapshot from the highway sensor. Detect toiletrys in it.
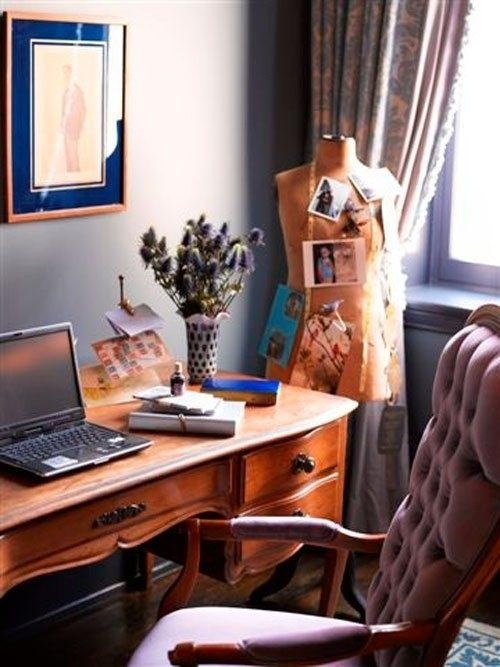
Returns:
[169,361,187,396]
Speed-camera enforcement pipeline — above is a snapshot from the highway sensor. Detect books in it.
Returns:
[200,375,282,404]
[126,384,247,436]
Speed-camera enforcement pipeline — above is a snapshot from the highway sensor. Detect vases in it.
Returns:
[184,312,231,384]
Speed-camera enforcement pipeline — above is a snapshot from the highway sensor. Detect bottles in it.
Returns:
[170,361,185,397]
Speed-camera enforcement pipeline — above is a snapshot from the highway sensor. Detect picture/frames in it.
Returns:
[3,10,133,223]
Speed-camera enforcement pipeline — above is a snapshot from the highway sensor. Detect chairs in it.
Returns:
[123,304,500,666]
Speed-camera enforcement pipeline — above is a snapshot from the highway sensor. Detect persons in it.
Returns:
[270,132,407,407]
[316,246,334,283]
[315,178,333,214]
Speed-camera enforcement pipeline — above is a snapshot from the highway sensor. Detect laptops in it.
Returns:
[0,322,154,478]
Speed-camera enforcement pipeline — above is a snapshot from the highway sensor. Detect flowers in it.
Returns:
[139,213,266,316]
